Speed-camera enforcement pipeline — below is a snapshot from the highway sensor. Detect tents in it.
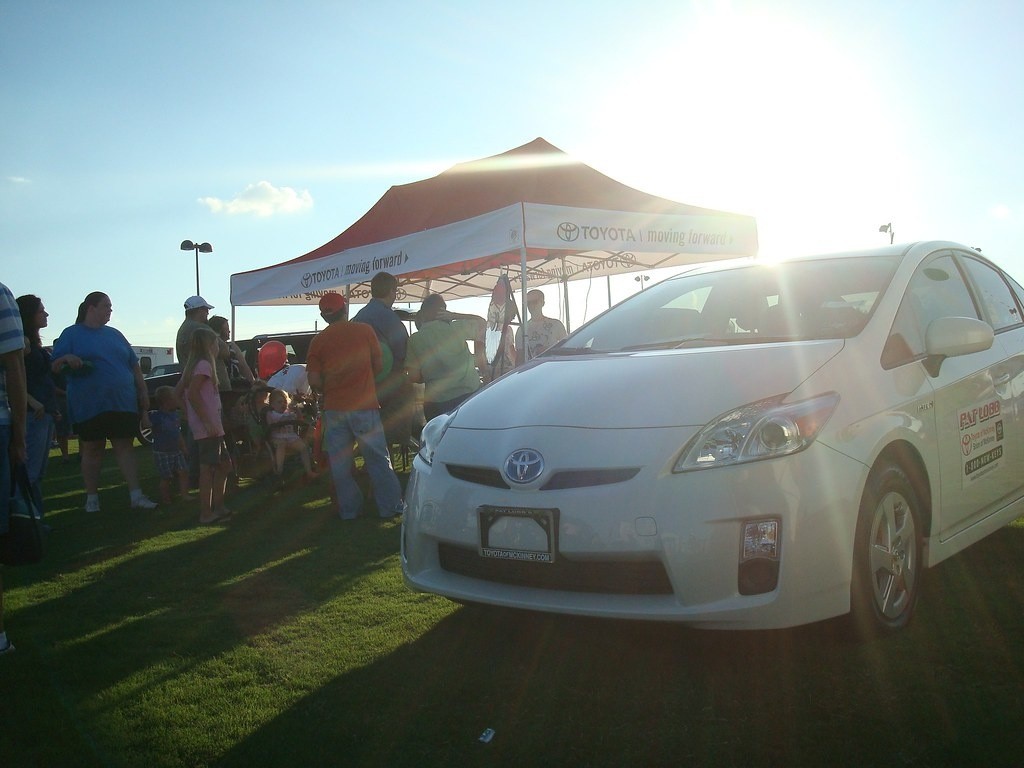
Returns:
[230,136,759,343]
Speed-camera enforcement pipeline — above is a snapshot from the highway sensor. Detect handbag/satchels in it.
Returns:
[0,461,44,564]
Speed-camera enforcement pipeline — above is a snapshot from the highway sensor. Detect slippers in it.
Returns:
[196,516,231,524]
[224,509,242,517]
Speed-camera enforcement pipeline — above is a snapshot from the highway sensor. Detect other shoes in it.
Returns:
[338,508,356,520]
[0,641,16,653]
[379,499,404,518]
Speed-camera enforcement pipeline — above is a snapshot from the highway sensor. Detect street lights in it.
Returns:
[180,239,212,296]
[879,224,892,245]
[632,274,653,290]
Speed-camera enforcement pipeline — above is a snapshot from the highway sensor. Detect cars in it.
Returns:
[397,239,1023,636]
[134,363,180,449]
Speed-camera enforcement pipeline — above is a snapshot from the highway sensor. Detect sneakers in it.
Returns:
[130,495,160,510]
[85,500,100,512]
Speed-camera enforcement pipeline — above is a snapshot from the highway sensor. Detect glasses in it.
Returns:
[528,299,542,306]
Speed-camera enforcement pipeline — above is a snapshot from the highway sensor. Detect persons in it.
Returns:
[405,294,487,422]
[209,317,255,382]
[144,386,186,489]
[305,292,404,520]
[515,289,567,369]
[266,389,320,480]
[353,271,409,445]
[176,296,238,493]
[12,295,93,531]
[50,292,158,512]
[182,328,232,523]
[0,281,27,533]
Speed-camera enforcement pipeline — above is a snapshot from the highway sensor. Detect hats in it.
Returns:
[319,292,345,313]
[185,296,215,311]
